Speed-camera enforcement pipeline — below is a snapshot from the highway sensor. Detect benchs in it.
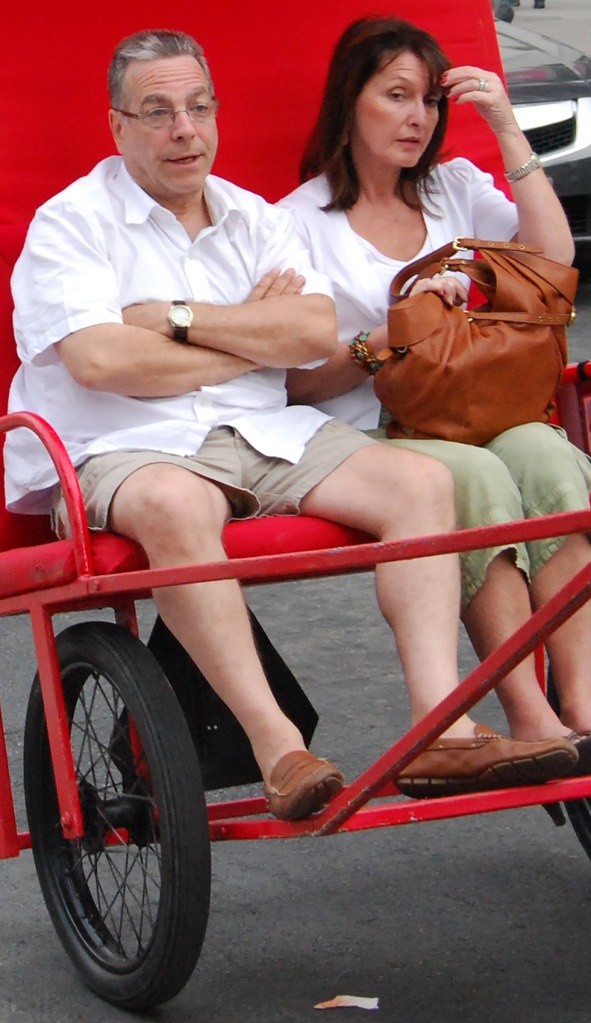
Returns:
[1,360,591,792]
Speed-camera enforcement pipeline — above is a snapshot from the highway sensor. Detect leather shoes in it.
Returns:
[266,755,344,821]
[393,722,578,797]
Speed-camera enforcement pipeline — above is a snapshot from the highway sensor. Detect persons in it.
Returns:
[273,13,591,776]
[4,30,579,819]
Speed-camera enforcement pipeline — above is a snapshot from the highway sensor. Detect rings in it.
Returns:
[479,79,485,90]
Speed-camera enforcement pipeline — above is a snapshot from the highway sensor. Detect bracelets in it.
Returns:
[349,331,380,375]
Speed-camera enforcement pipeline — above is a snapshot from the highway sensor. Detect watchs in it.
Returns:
[504,150,541,184]
[168,299,193,344]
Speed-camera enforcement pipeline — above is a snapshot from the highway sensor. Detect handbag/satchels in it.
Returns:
[373,234,577,447]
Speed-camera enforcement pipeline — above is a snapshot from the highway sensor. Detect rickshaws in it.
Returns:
[1,1,591,1005]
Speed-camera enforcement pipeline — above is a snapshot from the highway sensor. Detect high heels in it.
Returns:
[540,729,591,826]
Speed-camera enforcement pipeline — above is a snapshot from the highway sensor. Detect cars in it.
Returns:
[493,5,591,243]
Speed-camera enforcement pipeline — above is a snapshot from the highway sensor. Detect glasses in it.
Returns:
[110,95,221,130]
